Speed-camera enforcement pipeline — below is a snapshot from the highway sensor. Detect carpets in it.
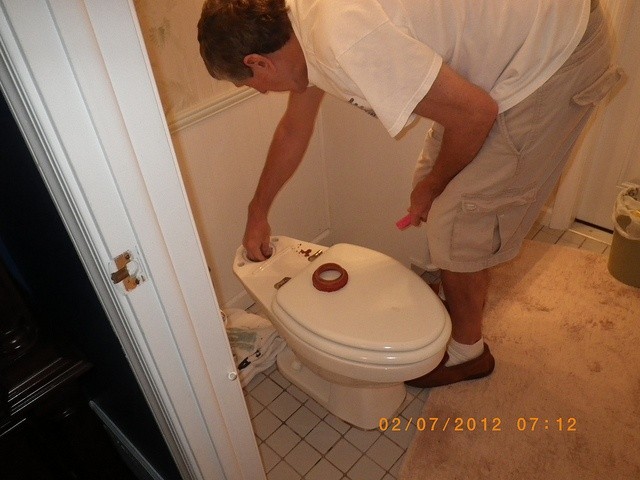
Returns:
[398,237,639,479]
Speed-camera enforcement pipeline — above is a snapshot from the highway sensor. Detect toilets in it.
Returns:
[232,234,453,431]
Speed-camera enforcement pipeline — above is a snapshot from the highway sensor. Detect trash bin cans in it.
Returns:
[607,186,640,288]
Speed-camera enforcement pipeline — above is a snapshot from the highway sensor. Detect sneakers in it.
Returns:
[405,343,495,388]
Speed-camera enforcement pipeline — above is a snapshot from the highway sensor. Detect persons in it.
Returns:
[198,2,622,388]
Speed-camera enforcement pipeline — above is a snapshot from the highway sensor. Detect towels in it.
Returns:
[220,308,288,389]
